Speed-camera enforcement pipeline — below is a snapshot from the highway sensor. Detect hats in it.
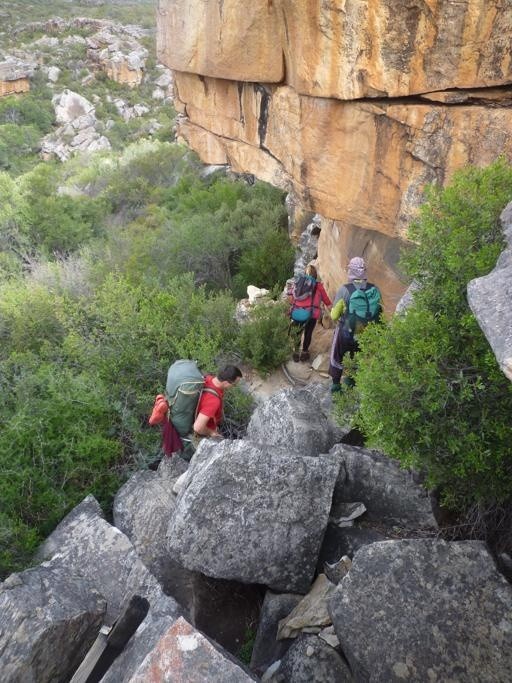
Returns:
[349,257,366,274]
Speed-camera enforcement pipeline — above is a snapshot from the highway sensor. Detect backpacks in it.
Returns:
[337,283,382,348]
[165,359,218,437]
[289,273,318,324]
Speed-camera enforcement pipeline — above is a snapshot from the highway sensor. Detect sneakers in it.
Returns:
[294,352,309,362]
[331,377,354,394]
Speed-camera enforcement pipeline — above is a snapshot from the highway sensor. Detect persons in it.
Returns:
[329,257,385,391]
[289,265,332,362]
[172,365,243,496]
